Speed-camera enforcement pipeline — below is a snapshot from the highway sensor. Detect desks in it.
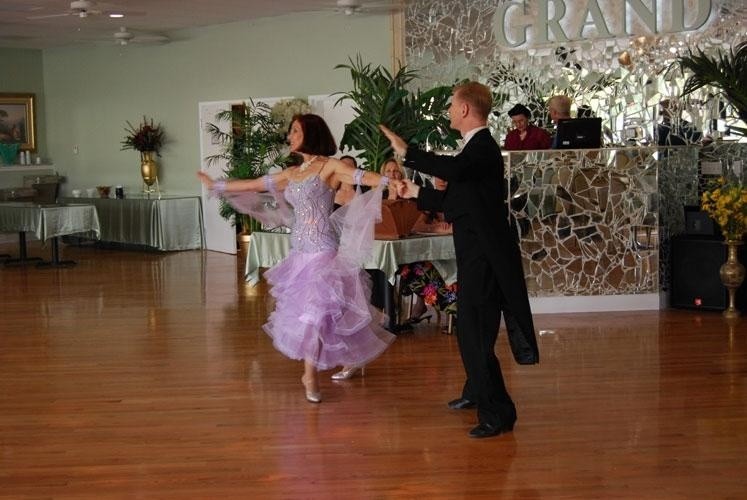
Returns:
[0,186,33,202]
[242,226,460,336]
[0,201,99,268]
[55,196,206,256]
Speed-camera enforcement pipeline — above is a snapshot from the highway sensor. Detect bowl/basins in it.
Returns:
[86,187,96,198]
[96,184,111,200]
[72,188,83,198]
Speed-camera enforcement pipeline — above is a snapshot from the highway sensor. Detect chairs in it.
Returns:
[38,177,65,202]
[29,181,57,206]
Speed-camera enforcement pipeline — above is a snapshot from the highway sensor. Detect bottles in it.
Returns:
[36,157,41,164]
[19,150,31,165]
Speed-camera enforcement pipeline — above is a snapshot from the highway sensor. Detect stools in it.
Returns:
[406,286,456,335]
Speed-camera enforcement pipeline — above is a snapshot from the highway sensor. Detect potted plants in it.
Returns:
[120,114,164,186]
[199,97,292,275]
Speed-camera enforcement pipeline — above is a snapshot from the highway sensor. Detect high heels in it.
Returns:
[301,376,323,403]
[403,315,433,326]
[330,362,366,381]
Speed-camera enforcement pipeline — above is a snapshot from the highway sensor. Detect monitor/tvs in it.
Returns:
[554,117,602,149]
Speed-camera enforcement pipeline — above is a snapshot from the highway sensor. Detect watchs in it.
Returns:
[548,95,571,149]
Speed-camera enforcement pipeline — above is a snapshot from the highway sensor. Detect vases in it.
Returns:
[719,240,747,319]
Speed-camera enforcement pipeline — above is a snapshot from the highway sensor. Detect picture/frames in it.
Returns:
[0,92,37,154]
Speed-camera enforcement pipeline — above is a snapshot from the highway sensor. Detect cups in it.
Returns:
[115,185,123,199]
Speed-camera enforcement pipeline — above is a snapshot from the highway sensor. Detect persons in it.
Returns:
[379,81,538,437]
[331,154,370,214]
[195,113,405,402]
[504,103,551,150]
[403,175,459,329]
[370,158,406,308]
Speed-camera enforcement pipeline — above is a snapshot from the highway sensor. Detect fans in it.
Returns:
[25,1,147,21]
[73,26,170,48]
[290,0,405,21]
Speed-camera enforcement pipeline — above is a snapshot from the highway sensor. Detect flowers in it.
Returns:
[695,173,747,246]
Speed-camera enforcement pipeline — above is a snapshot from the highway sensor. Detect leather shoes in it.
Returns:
[469,421,514,438]
[448,396,477,410]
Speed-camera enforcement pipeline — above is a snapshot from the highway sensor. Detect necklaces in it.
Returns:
[299,155,317,171]
